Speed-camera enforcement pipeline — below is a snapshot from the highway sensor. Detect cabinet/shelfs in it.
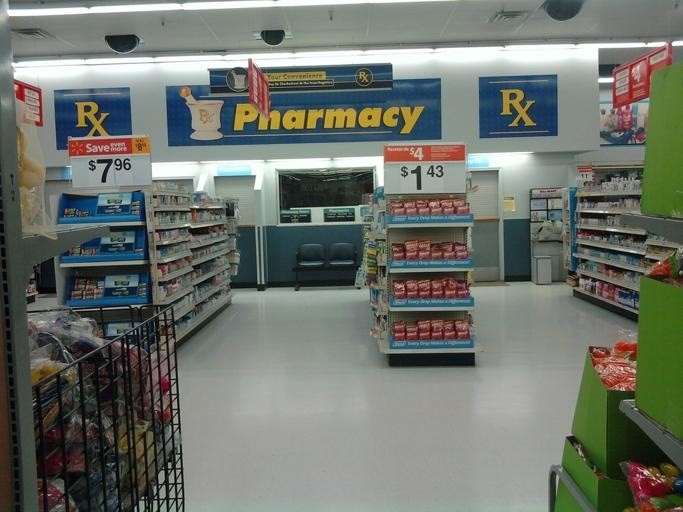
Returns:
[371,186,484,367]
[548,61,683,512]
[0,183,233,512]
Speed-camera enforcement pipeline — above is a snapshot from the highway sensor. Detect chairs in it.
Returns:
[291,243,362,291]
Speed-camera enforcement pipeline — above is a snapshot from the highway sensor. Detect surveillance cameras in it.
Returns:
[544,0,587,22]
[259,30,285,46]
[104,35,140,56]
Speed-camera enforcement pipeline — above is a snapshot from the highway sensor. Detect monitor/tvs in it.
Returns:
[275,167,374,211]
